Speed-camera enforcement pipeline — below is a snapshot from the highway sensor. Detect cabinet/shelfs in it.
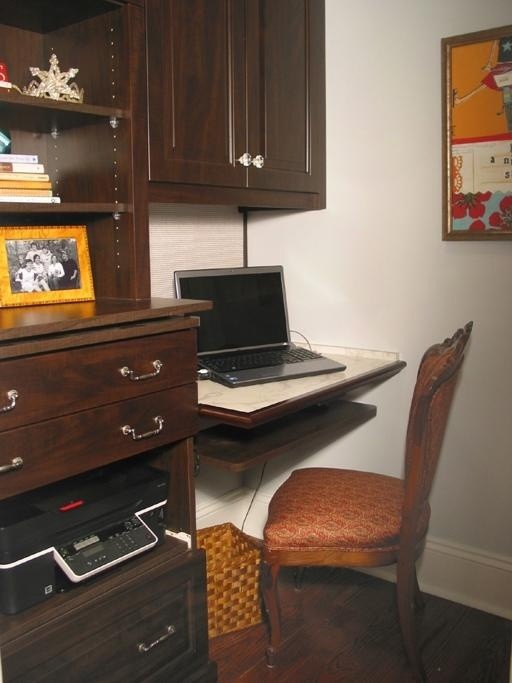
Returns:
[0,0,222,682]
[143,0,326,211]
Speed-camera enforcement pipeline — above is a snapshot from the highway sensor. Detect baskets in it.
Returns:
[196,522,268,639]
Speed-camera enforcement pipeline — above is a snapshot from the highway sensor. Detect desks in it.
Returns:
[195,340,407,472]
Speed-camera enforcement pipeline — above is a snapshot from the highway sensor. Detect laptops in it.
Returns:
[171,264,348,390]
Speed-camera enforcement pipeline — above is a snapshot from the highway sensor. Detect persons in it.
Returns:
[47,255,65,291]
[39,246,53,270]
[16,259,35,292]
[59,251,78,289]
[32,255,51,292]
[10,255,26,291]
[25,243,50,262]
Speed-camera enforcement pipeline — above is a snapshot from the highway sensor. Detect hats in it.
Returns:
[60,249,69,255]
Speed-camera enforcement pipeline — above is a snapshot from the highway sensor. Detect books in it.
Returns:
[0,173,49,181]
[0,163,45,174]
[0,189,52,197]
[0,182,52,189]
[0,154,38,164]
[0,196,61,203]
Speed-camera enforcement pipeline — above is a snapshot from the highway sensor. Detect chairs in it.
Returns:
[259,323,475,683]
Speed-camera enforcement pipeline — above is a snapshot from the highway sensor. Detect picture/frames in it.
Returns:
[440,23,512,243]
[1,225,98,307]
